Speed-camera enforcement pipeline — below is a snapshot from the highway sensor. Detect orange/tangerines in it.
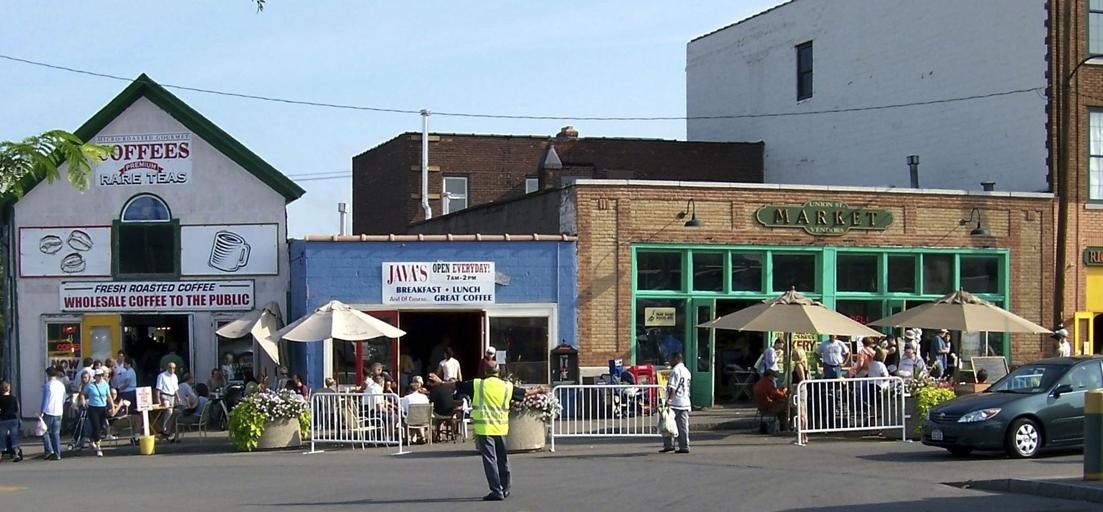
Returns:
[6,421,25,463]
[600,365,652,417]
[62,395,90,452]
[220,384,247,431]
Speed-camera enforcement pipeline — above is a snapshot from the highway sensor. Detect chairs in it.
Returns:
[674,448,690,453]
[659,446,675,453]
[482,492,504,502]
[88,442,96,448]
[42,450,53,459]
[50,454,61,459]
[502,489,512,498]
[97,448,102,457]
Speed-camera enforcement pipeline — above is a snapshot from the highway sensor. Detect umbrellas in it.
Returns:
[865,285,1055,397]
[694,286,887,438]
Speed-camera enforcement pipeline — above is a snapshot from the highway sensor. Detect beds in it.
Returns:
[210,302,290,391]
[694,286,887,438]
[269,299,408,447]
[865,285,1055,397]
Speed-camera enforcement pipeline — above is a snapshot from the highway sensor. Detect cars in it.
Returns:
[209,230,252,272]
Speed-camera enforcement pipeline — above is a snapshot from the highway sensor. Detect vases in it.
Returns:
[910,376,956,435]
[509,384,562,422]
[226,389,311,451]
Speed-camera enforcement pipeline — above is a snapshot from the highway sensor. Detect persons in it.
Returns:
[429,362,550,500]
[0,380,21,463]
[753,328,959,444]
[160,346,184,385]
[478,346,496,379]
[41,347,136,459]
[156,362,181,432]
[273,368,291,392]
[1070,368,1086,391]
[221,354,234,379]
[178,373,199,408]
[236,357,250,380]
[242,374,264,396]
[207,368,227,394]
[158,382,209,443]
[351,346,464,447]
[1055,329,1071,357]
[1050,329,1066,357]
[659,352,691,453]
[325,377,334,389]
[975,369,989,384]
[292,373,308,400]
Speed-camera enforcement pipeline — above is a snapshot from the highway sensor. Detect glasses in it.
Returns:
[678,198,705,227]
[959,207,989,236]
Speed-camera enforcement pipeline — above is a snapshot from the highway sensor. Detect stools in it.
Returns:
[161,393,175,397]
[823,363,839,367]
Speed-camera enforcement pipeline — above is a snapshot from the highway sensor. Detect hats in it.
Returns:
[924,357,1101,459]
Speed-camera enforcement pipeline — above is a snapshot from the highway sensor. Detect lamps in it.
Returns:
[404,402,434,447]
[458,396,473,439]
[434,400,464,444]
[175,400,212,443]
[351,400,374,440]
[343,405,389,450]
[105,415,136,450]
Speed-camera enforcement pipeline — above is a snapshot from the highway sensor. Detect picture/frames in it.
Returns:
[134,404,169,442]
[726,370,756,401]
[211,392,225,426]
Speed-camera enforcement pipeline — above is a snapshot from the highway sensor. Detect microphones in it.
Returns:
[759,413,781,435]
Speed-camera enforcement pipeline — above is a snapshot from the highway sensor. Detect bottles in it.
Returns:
[95,368,104,375]
[1054,329,1069,337]
[486,346,496,355]
[483,361,500,373]
[938,327,949,334]
[904,329,916,340]
